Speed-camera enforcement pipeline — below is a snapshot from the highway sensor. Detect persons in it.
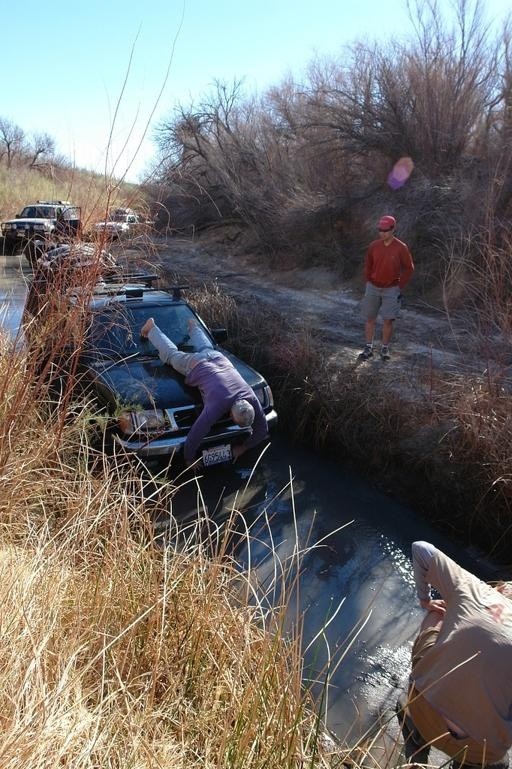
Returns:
[139,316,270,475]
[395,538,512,769]
[357,215,415,361]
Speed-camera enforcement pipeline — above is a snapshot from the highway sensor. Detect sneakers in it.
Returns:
[379,346,391,361]
[358,346,374,361]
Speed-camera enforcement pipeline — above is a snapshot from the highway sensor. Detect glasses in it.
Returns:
[377,226,393,232]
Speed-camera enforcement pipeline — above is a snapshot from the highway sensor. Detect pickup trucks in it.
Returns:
[93,210,155,239]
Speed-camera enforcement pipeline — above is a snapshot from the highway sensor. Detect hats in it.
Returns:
[375,216,395,230]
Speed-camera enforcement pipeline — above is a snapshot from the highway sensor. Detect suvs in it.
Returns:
[0,196,83,264]
[21,263,280,480]
